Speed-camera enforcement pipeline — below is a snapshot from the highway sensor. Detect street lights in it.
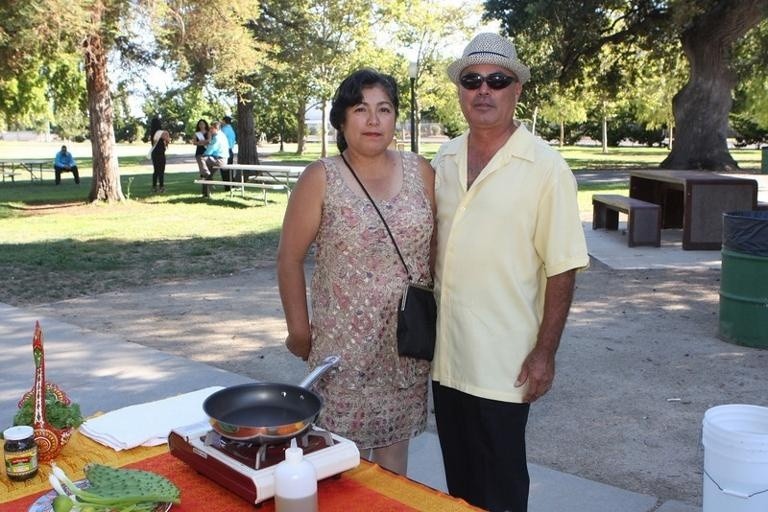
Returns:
[407,61,418,153]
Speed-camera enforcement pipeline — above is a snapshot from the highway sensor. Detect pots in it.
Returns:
[203,356,340,445]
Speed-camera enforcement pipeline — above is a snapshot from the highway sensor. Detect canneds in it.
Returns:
[3,425,39,482]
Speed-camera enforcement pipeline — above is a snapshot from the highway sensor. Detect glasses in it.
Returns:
[458,71,514,90]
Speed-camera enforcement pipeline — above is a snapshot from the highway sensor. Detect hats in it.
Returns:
[445,32,531,87]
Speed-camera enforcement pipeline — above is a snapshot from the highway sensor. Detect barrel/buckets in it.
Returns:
[702,403,767,512]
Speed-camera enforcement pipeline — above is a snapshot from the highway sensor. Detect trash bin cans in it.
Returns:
[761,146,768,175]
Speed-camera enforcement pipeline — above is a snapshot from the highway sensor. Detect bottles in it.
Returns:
[272,438,318,511]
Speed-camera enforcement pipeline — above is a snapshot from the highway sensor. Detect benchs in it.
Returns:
[0,159,64,185]
[591,193,662,248]
[754,200,768,212]
[193,163,306,205]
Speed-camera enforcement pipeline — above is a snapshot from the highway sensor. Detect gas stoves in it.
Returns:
[168,419,360,507]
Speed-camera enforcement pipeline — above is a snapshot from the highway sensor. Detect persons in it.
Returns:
[193,119,212,155]
[275,67,439,478]
[219,116,237,192]
[150,118,170,193]
[196,122,228,196]
[53,145,80,186]
[427,33,589,511]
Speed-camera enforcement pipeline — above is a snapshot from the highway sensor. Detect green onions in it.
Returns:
[48,461,181,512]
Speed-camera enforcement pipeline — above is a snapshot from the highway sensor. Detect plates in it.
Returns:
[28,479,172,511]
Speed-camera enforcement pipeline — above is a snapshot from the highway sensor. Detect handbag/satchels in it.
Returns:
[396,281,438,364]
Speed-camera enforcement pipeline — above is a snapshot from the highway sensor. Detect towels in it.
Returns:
[76,386,232,454]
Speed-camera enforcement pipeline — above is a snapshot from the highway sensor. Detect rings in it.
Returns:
[534,392,541,398]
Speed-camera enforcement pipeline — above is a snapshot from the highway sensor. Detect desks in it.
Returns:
[628,169,758,251]
[0,384,494,512]
